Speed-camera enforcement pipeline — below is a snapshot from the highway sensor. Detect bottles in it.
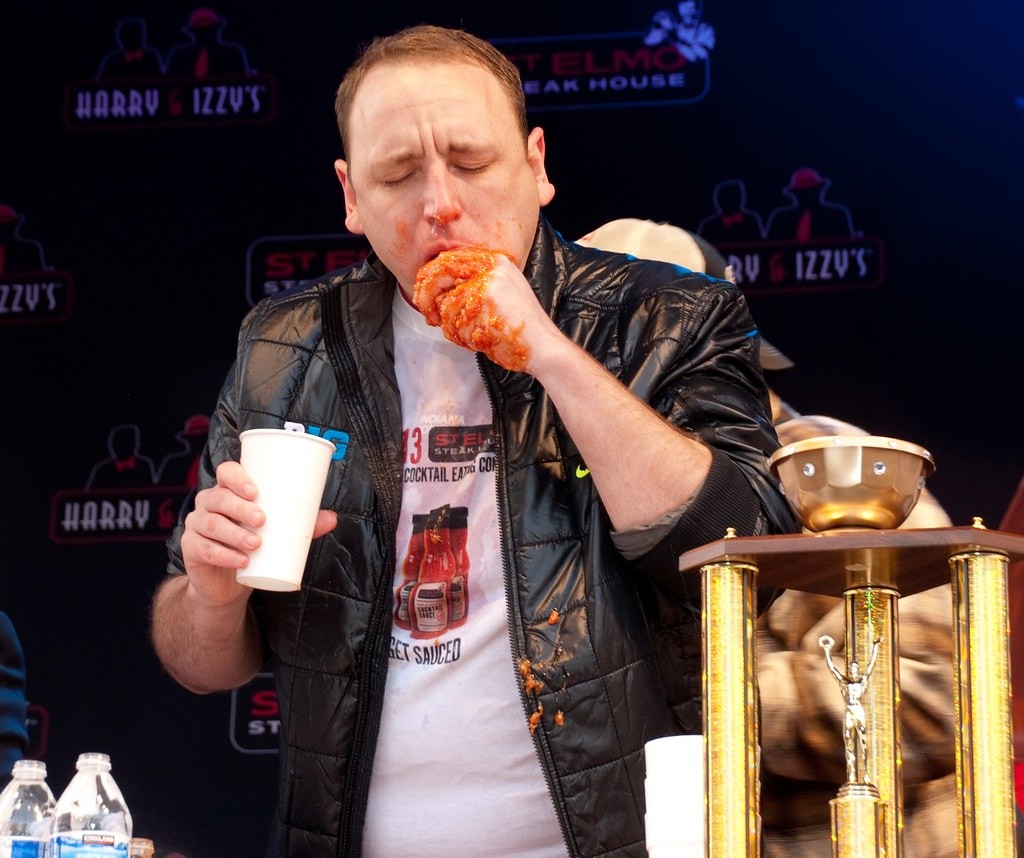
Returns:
[44,752,132,858]
[394,503,469,641]
[0,759,57,858]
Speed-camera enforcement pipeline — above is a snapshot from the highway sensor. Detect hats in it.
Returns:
[574,217,796,371]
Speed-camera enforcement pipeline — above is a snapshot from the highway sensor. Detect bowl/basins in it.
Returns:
[768,434,936,533]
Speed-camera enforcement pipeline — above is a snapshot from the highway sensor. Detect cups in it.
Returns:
[233,427,338,596]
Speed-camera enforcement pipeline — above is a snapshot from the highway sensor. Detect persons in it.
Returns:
[153,26,805,858]
[562,218,961,858]
